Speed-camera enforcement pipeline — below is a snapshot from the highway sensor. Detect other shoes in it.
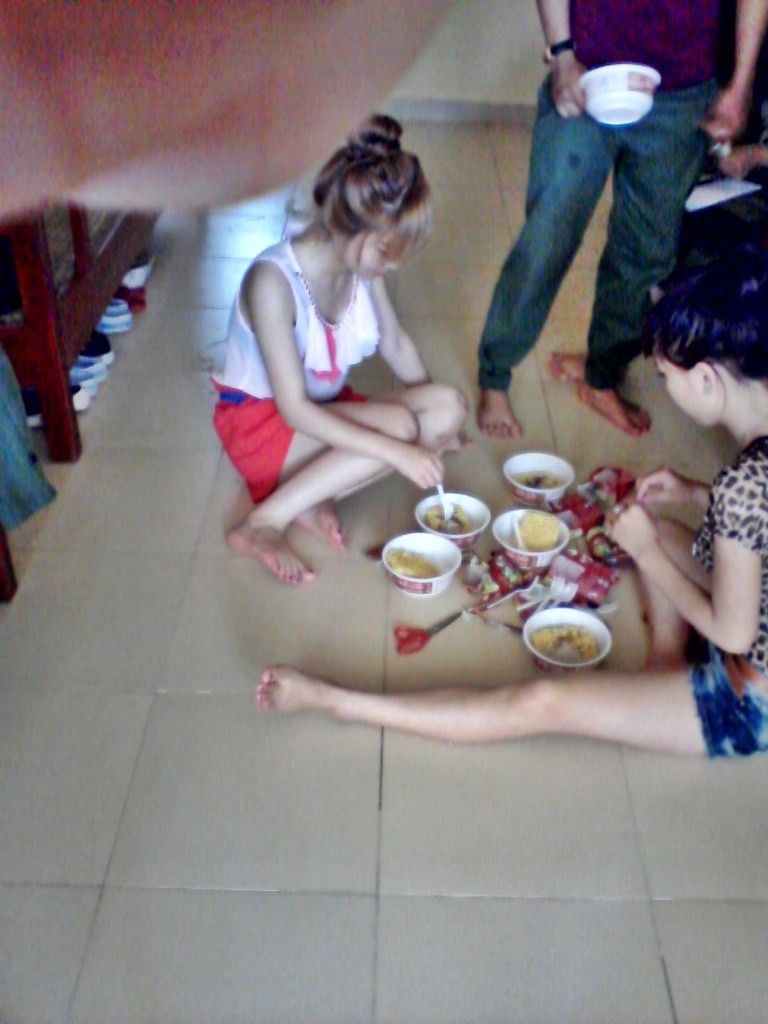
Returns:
[25,251,154,428]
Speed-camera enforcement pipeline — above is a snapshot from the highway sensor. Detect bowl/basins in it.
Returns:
[578,61,662,126]
[491,509,571,576]
[413,492,491,562]
[503,452,575,507]
[522,608,614,677]
[381,532,462,598]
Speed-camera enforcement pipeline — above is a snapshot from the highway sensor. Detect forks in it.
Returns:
[437,485,453,521]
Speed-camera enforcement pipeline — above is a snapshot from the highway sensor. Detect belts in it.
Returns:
[721,651,768,696]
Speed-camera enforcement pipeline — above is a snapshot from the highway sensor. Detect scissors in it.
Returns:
[395,610,463,655]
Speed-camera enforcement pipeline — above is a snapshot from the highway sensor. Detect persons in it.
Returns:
[476,0,768,435]
[257,261,768,760]
[213,112,468,584]
[0,0,460,218]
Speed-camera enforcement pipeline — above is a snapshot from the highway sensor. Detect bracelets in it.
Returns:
[544,38,577,63]
